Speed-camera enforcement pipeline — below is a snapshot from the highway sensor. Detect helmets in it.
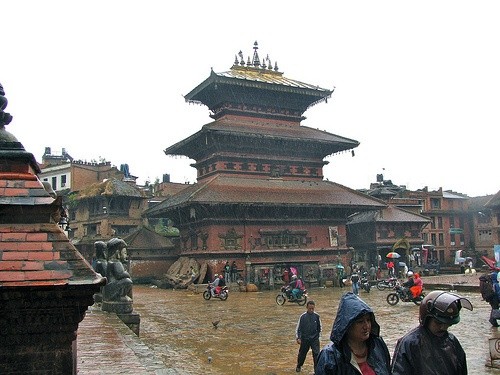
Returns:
[419,290,461,333]
[408,271,414,277]
[214,274,218,277]
[291,275,297,280]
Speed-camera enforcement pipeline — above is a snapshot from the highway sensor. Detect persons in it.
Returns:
[401,271,423,298]
[283,268,294,286]
[372,252,394,275]
[460,259,472,273]
[224,261,237,281]
[295,301,322,372]
[210,273,225,297]
[187,265,197,285]
[338,262,375,296]
[400,253,419,270]
[488,265,500,327]
[287,276,304,301]
[314,291,391,375]
[94,238,134,302]
[391,290,468,375]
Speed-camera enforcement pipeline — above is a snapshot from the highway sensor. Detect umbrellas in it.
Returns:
[290,267,298,274]
[335,265,344,269]
[386,252,401,258]
[456,257,472,262]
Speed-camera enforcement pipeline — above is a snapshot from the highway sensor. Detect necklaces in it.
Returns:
[350,347,367,358]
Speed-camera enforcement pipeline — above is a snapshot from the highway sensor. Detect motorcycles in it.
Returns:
[375,273,401,291]
[386,281,426,306]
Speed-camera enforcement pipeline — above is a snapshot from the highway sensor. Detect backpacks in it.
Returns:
[478,273,499,301]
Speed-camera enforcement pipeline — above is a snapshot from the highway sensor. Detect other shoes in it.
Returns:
[296,366,300,372]
[489,319,499,327]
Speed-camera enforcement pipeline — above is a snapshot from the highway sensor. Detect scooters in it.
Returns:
[358,271,371,294]
[276,285,308,306]
[202,281,229,301]
